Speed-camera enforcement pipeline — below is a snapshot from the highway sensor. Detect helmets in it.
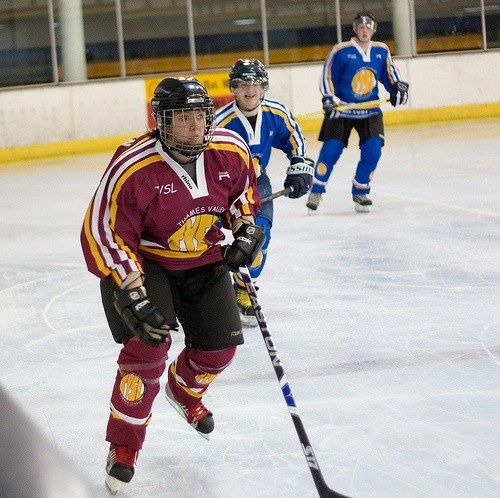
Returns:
[353,11,377,33]
[229,58,269,93]
[151,75,213,139]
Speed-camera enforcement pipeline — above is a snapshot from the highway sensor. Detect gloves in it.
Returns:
[285,156,314,198]
[390,81,409,107]
[113,284,172,350]
[221,224,266,273]
[323,95,342,120]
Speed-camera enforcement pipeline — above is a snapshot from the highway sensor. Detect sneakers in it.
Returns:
[105,443,136,495]
[353,194,373,212]
[306,192,321,214]
[165,381,214,441]
[234,282,259,328]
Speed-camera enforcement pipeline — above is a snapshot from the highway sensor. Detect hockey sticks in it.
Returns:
[236,264,355,498]
[295,98,395,128]
[258,185,298,205]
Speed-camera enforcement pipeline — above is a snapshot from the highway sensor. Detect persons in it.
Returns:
[209,59,314,327]
[307,11,409,215]
[82,77,266,494]
